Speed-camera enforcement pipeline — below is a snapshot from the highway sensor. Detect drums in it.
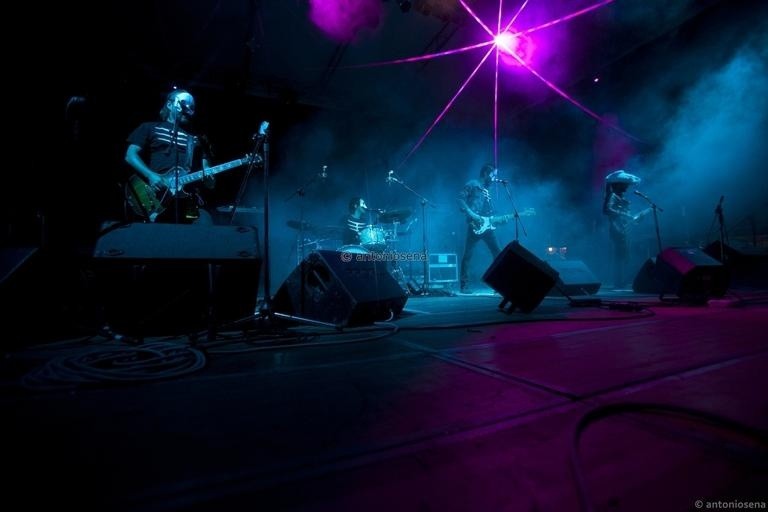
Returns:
[337,243,371,254]
[357,224,387,252]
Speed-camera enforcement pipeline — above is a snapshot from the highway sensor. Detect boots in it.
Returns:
[461,279,472,294]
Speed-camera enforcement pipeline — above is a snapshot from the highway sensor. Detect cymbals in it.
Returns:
[312,225,346,240]
[287,221,314,230]
[377,210,411,223]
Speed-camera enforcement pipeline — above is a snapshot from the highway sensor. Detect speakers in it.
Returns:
[91,223,259,341]
[632,256,657,294]
[548,258,602,301]
[271,251,409,329]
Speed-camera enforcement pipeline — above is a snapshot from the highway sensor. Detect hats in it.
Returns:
[606,170,641,184]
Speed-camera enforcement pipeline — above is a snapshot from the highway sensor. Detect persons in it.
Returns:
[455,162,504,293]
[338,197,368,246]
[601,169,641,287]
[123,88,214,225]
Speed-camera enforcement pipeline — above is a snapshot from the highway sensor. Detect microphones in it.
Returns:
[321,164,328,180]
[633,189,648,200]
[490,176,507,185]
[174,96,194,118]
[386,168,394,187]
[253,122,269,141]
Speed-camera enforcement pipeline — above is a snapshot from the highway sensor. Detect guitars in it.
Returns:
[611,207,654,234]
[125,153,263,217]
[468,208,537,239]
